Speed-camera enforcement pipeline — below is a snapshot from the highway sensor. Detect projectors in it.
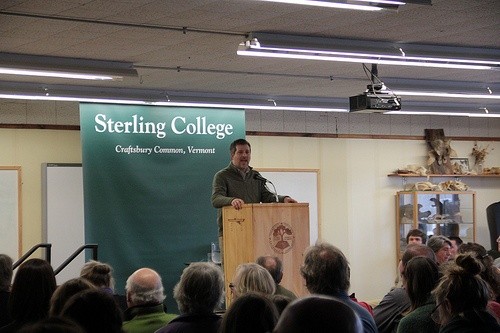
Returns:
[348,94,402,109]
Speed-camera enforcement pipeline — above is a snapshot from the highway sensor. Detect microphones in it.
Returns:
[253,173,270,182]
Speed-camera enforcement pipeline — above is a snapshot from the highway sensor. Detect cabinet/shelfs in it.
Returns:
[394,191,476,279]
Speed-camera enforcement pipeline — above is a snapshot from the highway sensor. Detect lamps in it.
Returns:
[0,0,500,119]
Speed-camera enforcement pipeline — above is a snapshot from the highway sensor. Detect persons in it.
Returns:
[0,228,500,333]
[210,139,296,270]
